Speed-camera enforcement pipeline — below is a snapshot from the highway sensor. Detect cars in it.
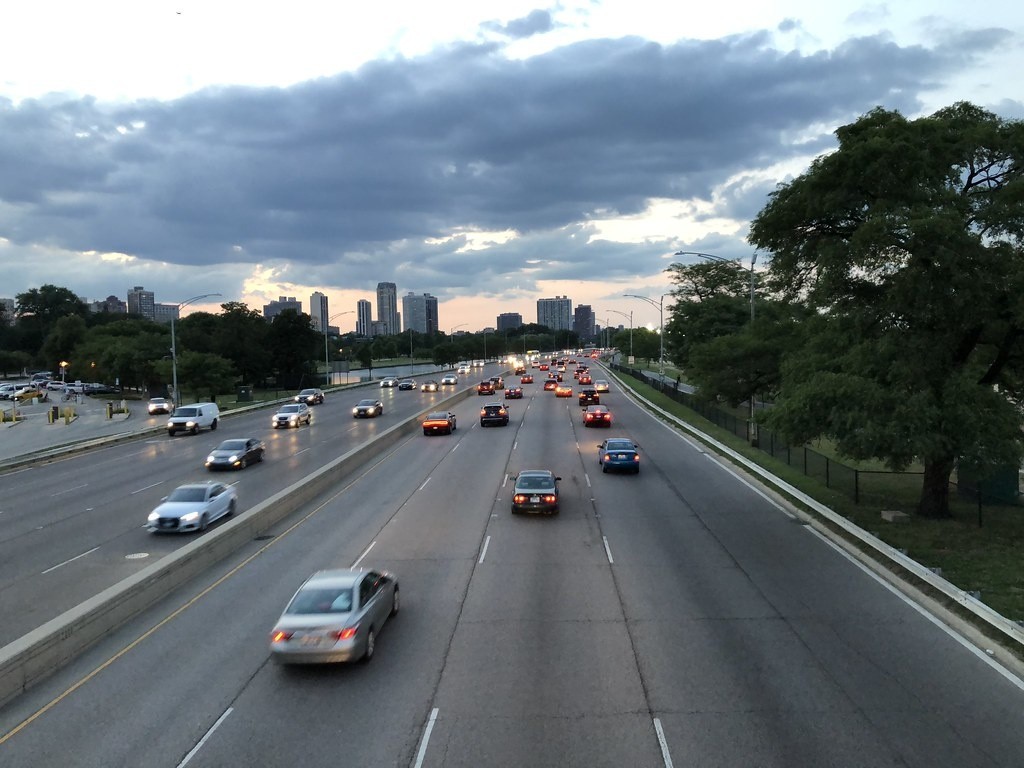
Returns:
[0,371,107,402]
[381,376,399,388]
[399,378,418,390]
[509,469,562,516]
[295,388,324,405]
[205,437,266,472]
[582,404,612,428]
[505,386,523,399]
[422,410,456,435]
[147,397,169,415]
[457,360,485,373]
[148,480,238,533]
[478,377,505,395]
[594,379,609,393]
[578,388,600,406]
[352,398,382,418]
[271,402,312,428]
[480,402,510,427]
[422,379,439,392]
[270,567,400,666]
[442,374,458,385]
[498,348,602,397]
[596,437,640,474]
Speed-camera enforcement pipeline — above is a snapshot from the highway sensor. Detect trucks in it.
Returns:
[166,403,220,437]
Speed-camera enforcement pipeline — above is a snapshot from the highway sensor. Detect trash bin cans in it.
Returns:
[237,386,254,401]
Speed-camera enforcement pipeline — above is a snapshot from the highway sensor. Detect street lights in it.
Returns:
[451,323,469,368]
[409,319,432,374]
[170,293,222,413]
[606,309,633,375]
[623,294,665,394]
[673,250,756,435]
[324,310,356,386]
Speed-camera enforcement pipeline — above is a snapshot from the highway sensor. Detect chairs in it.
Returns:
[542,482,550,488]
[522,483,529,489]
